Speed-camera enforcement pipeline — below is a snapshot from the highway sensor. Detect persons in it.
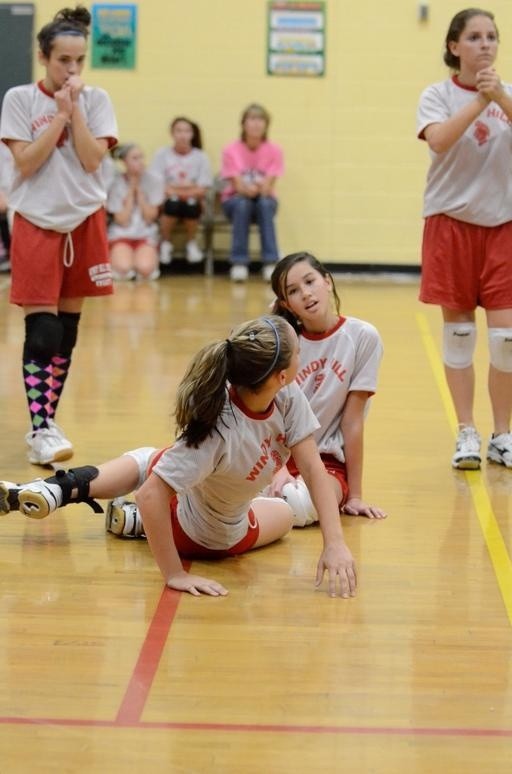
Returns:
[213,102,282,286]
[134,313,361,600]
[413,6,512,474]
[1,244,388,542]
[0,5,119,465]
[105,140,163,278]
[154,117,206,259]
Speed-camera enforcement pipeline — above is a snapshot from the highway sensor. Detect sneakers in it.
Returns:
[105,498,145,539]
[26,420,74,464]
[488,431,511,467]
[0,477,63,519]
[453,426,483,470]
[159,240,275,284]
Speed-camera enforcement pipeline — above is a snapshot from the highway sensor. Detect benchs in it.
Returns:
[145,214,265,278]
